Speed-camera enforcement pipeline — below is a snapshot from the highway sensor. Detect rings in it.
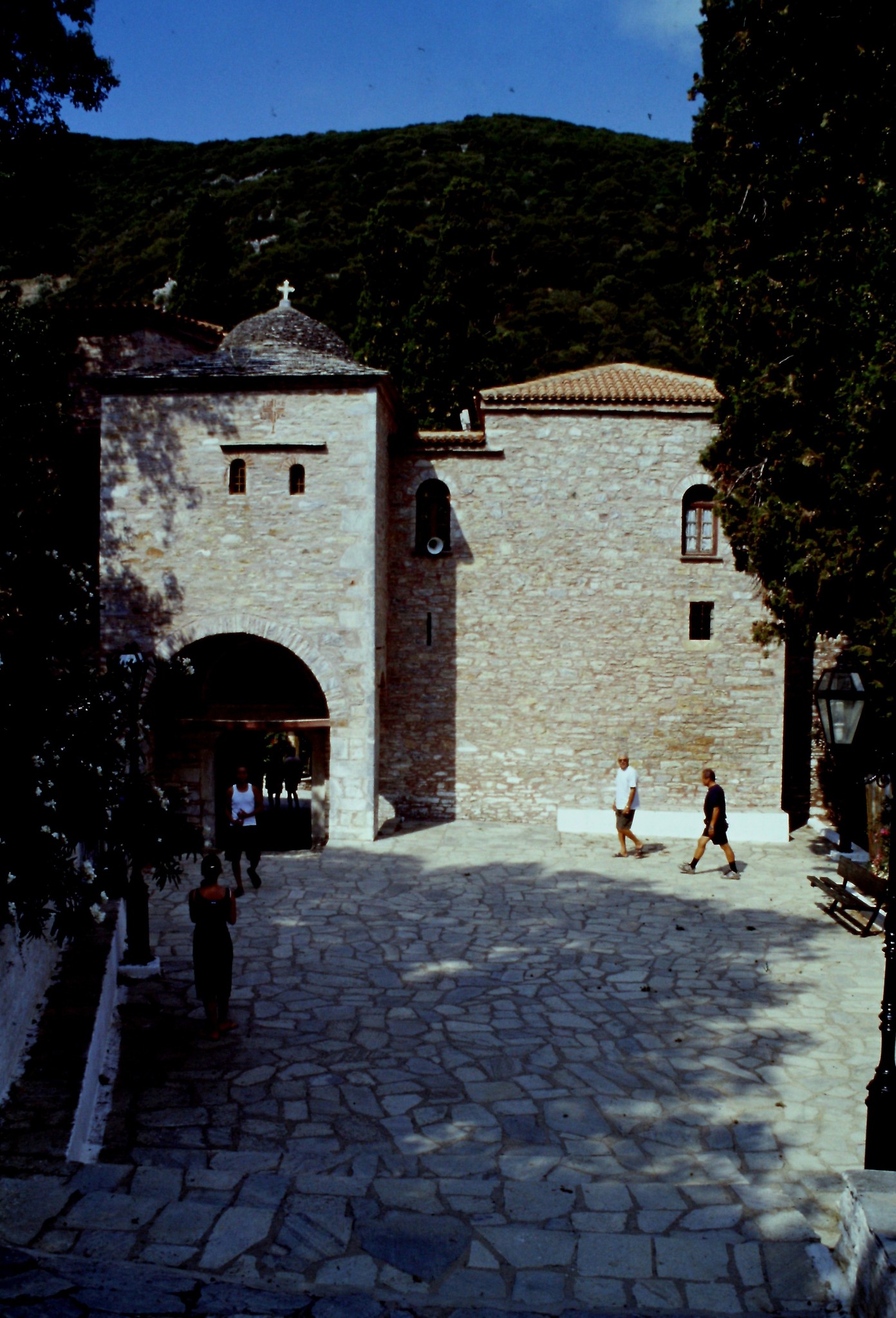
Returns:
[626,812,628,813]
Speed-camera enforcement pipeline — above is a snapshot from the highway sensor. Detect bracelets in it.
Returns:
[626,806,630,808]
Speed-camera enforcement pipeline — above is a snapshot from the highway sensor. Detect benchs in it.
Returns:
[807,855,889,936]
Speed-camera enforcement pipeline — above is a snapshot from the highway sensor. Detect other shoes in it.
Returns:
[211,1032,219,1040]
[219,1020,236,1029]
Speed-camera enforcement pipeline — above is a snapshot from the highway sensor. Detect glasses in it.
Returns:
[619,759,627,762]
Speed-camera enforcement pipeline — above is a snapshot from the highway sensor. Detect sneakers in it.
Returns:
[720,869,740,879]
[679,864,696,874]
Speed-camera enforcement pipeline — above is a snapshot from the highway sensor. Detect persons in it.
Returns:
[267,747,303,810]
[612,754,644,858]
[188,854,239,1038]
[678,769,740,880]
[225,766,264,898]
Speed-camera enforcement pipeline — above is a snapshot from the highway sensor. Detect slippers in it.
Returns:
[612,853,625,857]
[247,867,262,888]
[233,886,244,896]
[635,845,644,858]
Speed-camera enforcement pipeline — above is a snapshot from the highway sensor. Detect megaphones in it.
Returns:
[428,537,444,554]
